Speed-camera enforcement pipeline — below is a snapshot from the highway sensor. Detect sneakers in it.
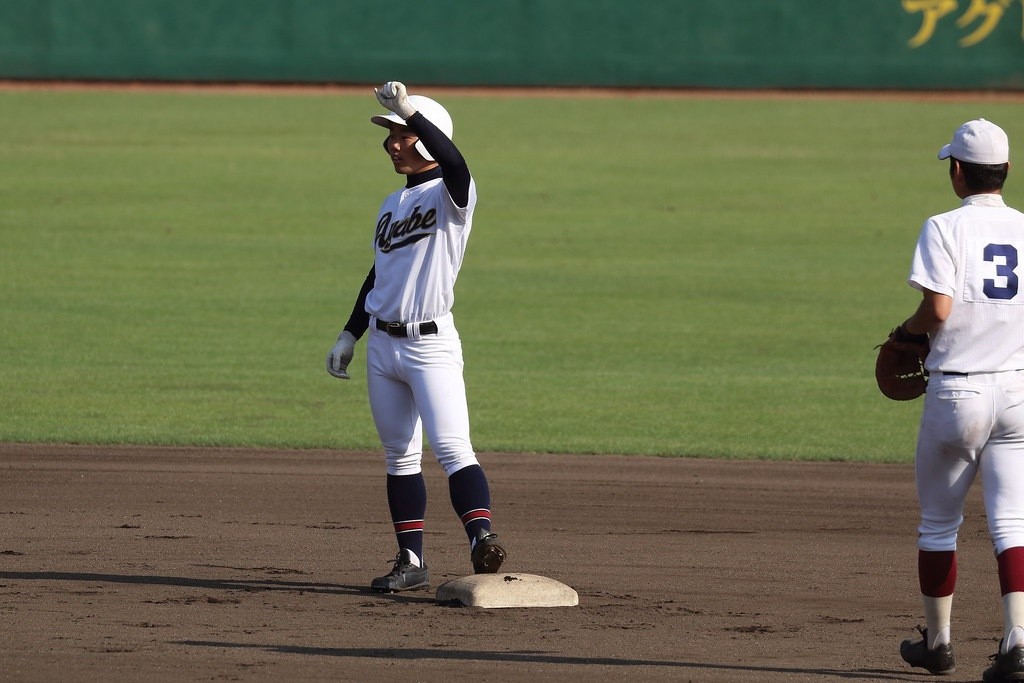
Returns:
[983,638,1024,682]
[371,548,429,594]
[471,528,506,574]
[900,625,956,675]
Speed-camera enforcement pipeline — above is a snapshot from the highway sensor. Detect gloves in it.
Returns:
[374,81,415,120]
[326,330,358,379]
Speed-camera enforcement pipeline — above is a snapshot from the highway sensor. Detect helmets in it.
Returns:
[371,95,453,162]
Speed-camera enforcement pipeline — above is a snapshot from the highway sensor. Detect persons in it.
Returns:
[327,81,507,592]
[875,118,1024,683]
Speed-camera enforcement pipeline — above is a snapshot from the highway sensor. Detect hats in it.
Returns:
[937,117,1009,164]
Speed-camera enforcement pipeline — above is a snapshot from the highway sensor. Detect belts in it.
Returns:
[368,315,437,338]
[943,371,967,375]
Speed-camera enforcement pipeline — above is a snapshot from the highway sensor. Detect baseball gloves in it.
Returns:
[873,320,929,402]
